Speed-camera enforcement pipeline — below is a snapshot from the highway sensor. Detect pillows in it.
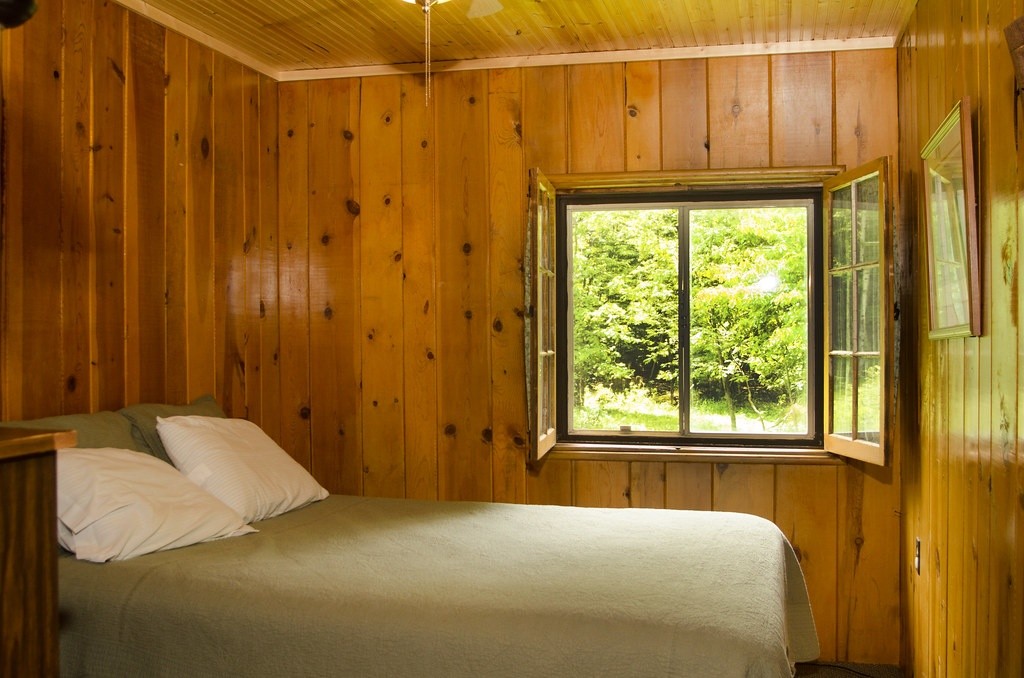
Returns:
[155,414,330,525]
[117,392,227,470]
[53,447,261,564]
[0,411,156,459]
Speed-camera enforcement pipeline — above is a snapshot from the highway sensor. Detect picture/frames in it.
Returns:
[915,95,982,341]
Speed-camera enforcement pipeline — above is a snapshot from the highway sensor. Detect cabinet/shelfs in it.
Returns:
[0,424,78,678]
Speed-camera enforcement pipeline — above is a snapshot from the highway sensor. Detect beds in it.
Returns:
[58,494,821,678]
